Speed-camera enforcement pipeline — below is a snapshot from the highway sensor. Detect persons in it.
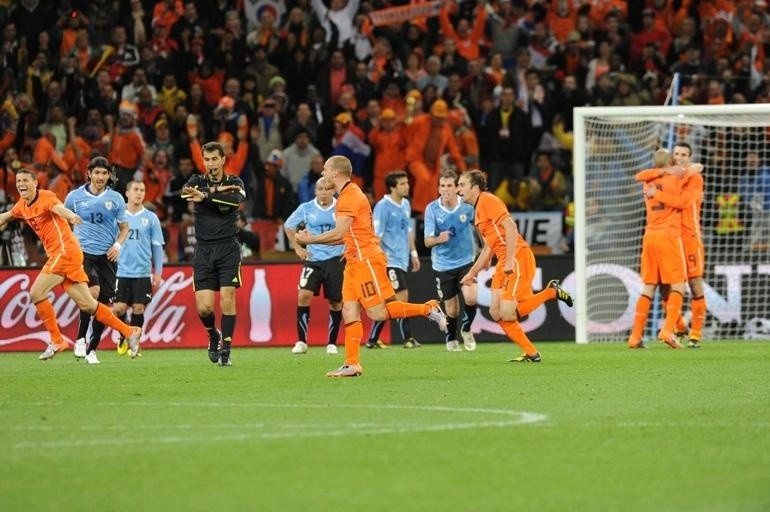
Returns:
[1,1,770,376]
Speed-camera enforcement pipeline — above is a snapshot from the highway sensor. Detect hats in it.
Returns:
[336,90,448,125]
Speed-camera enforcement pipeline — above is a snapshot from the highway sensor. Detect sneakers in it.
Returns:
[39,323,143,365]
[627,336,647,349]
[366,339,389,349]
[208,329,222,365]
[292,341,308,354]
[445,327,475,352]
[508,352,541,364]
[547,278,575,307]
[403,337,420,349]
[326,343,338,354]
[656,327,701,349]
[326,363,362,378]
[426,298,449,330]
[218,348,233,367]
